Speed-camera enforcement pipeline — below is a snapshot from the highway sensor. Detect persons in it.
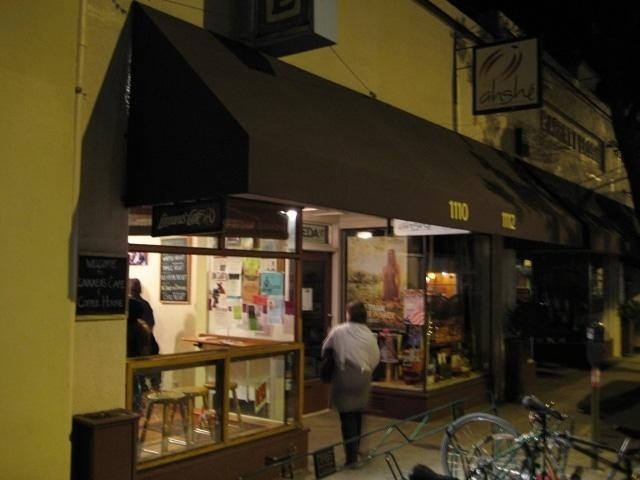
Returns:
[127,278,163,393]
[381,247,401,303]
[320,299,381,468]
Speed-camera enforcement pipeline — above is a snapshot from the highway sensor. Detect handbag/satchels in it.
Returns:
[320,349,335,383]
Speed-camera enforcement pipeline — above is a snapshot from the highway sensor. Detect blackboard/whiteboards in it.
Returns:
[159,236,192,304]
[76,250,128,320]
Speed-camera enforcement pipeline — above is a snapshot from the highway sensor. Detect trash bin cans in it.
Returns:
[508,336,529,402]
[71,408,141,480]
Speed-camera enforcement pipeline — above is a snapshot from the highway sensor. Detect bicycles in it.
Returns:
[440,394,640,480]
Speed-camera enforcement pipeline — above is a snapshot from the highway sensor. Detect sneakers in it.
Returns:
[340,462,358,469]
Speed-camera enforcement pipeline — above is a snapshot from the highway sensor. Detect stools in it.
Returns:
[140,381,245,450]
